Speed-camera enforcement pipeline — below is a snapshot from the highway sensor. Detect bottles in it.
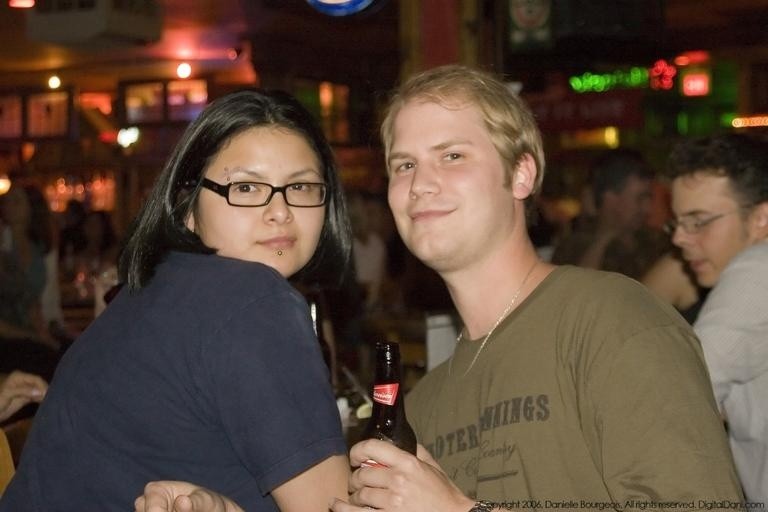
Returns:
[360,342,417,455]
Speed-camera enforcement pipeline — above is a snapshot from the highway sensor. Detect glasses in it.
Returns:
[661,204,754,235]
[196,177,331,208]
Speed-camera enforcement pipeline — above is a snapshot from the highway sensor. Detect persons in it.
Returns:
[3,61,767,511]
